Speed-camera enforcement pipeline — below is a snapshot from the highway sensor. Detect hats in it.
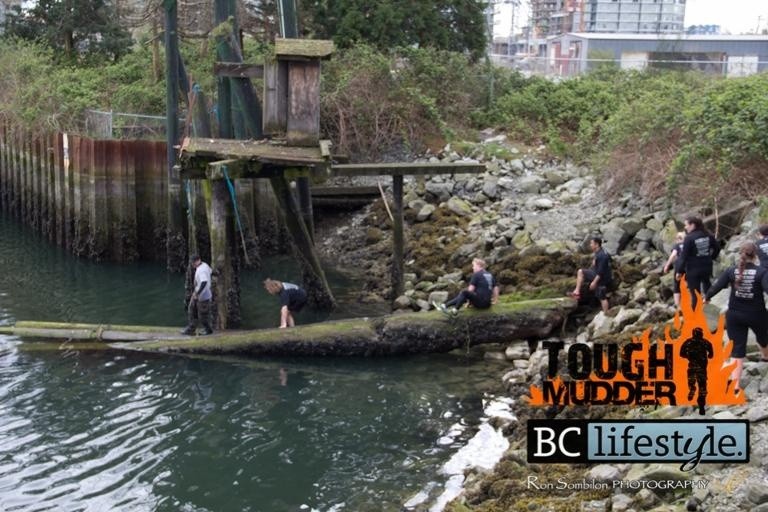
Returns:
[190,254,200,263]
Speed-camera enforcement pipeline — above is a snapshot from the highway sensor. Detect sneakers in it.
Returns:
[432,301,458,317]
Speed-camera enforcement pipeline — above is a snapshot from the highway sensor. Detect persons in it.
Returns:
[663,231,686,310]
[261,278,309,329]
[565,238,614,314]
[703,240,767,395]
[679,327,713,414]
[178,255,219,336]
[432,255,499,316]
[675,216,720,312]
[754,225,768,295]
